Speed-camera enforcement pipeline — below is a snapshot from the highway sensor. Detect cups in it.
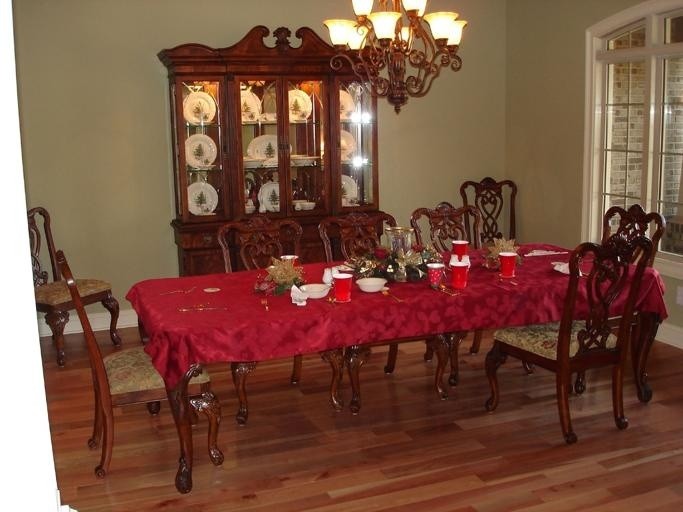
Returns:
[427,263,445,288]
[450,262,469,289]
[452,240,469,262]
[498,252,517,278]
[280,255,300,270]
[333,273,353,303]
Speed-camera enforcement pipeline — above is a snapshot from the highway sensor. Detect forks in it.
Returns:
[260,298,269,312]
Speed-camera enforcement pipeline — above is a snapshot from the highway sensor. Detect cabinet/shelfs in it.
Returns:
[156,26,398,277]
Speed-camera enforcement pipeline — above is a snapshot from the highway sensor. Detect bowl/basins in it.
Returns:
[300,202,315,210]
[355,277,387,293]
[243,159,267,168]
[299,284,330,299]
[183,90,356,125]
[290,157,320,165]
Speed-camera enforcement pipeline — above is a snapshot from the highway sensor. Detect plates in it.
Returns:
[342,174,358,207]
[197,165,217,168]
[185,134,217,168]
[194,121,215,124]
[258,182,280,213]
[195,213,216,216]
[187,183,218,214]
[247,134,292,167]
[340,130,355,160]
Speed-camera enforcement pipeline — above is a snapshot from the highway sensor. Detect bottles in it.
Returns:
[322,268,333,283]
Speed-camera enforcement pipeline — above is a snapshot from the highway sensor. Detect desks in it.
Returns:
[124,241,670,494]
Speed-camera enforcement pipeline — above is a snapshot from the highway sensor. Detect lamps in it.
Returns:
[321,1,468,114]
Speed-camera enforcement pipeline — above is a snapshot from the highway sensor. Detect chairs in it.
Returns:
[27,207,120,368]
[218,211,346,386]
[481,234,653,445]
[457,175,519,245]
[318,211,435,383]
[407,199,485,357]
[56,248,223,479]
[600,202,667,268]
